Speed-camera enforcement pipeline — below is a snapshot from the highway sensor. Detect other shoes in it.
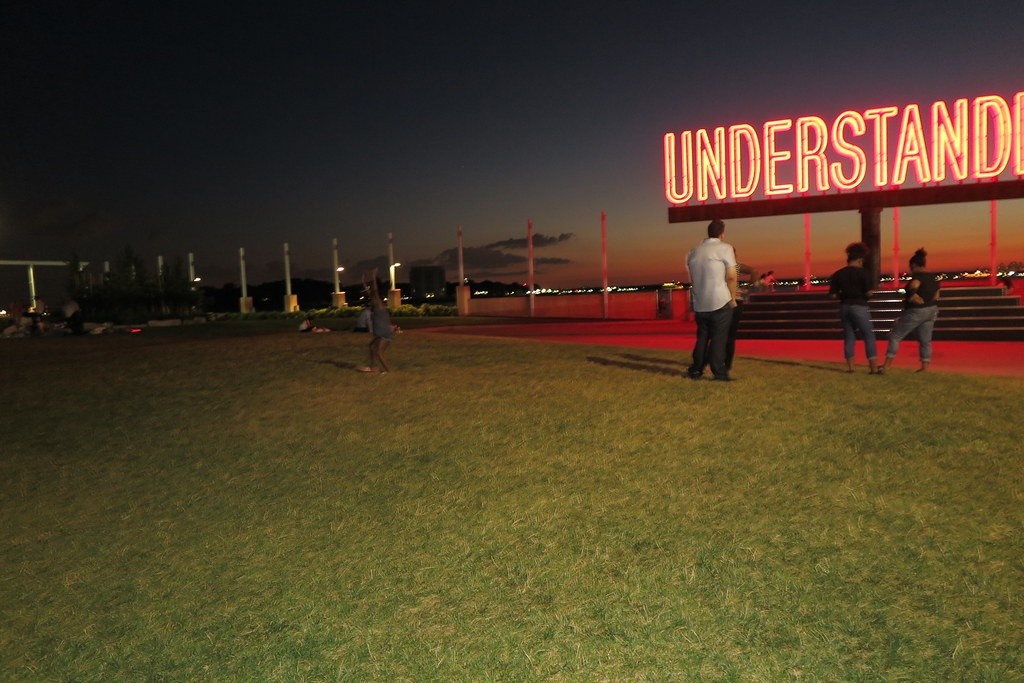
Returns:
[381,371,388,374]
[687,369,702,379]
[360,366,372,371]
[714,377,735,381]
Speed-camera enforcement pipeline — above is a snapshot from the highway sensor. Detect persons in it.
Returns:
[2,295,86,341]
[754,271,775,292]
[830,242,883,376]
[354,295,393,376]
[687,219,742,381]
[878,246,940,373]
[299,315,315,332]
